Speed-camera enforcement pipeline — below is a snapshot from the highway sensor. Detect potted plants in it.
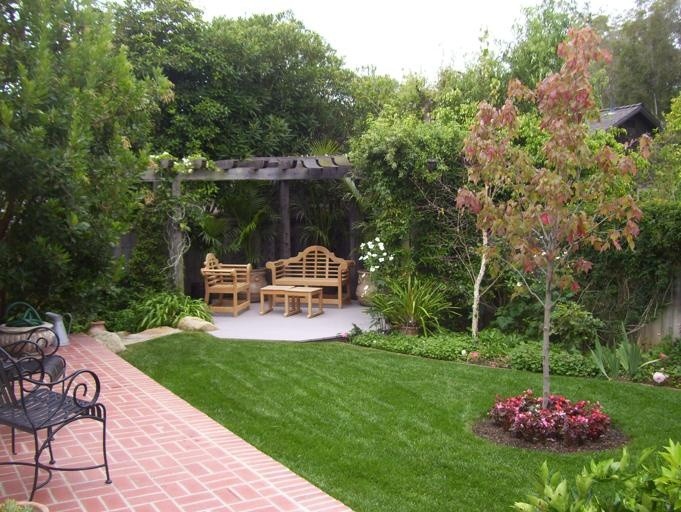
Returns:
[361,270,463,338]
[0,497,50,512]
[352,178,378,306]
[0,308,56,357]
[197,180,282,303]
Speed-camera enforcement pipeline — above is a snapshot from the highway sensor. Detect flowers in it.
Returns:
[358,237,394,272]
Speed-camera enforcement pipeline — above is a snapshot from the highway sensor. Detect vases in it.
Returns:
[88,320,106,337]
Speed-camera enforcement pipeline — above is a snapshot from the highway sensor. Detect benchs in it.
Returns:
[266,245,355,309]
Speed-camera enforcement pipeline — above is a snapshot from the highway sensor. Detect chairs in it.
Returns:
[200,253,251,317]
[0,346,112,501]
[0,327,67,441]
